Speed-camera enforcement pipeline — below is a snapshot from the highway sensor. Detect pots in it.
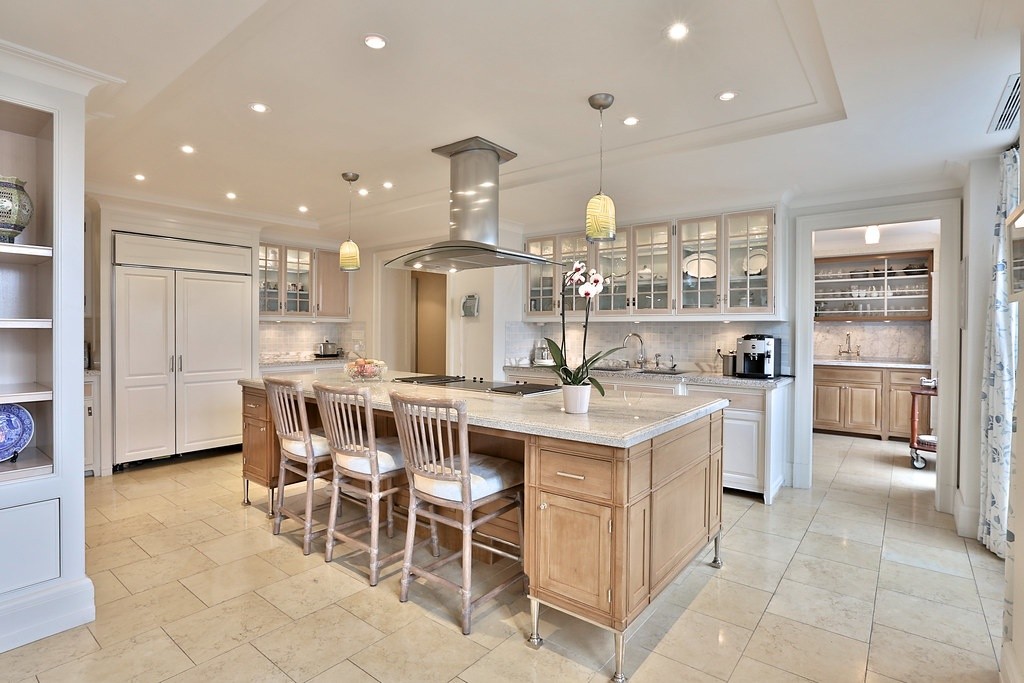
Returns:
[315,343,343,356]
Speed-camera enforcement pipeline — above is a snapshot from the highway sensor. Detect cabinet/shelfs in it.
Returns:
[0,96,96,656]
[812,251,934,322]
[685,385,766,495]
[813,365,931,441]
[909,385,938,468]
[106,231,254,466]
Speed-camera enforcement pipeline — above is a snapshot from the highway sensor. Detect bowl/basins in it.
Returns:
[850,270,868,278]
[903,264,928,275]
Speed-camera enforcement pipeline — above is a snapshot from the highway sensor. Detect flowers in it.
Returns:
[543,261,627,397]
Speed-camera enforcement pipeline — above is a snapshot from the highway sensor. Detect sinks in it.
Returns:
[638,367,688,378]
[589,364,630,376]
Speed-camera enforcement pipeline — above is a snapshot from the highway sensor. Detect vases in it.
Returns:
[562,383,591,415]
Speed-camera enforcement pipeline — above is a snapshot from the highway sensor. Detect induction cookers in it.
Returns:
[392,376,563,396]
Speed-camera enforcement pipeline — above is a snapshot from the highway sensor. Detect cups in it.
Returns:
[531,299,536,311]
[722,354,736,376]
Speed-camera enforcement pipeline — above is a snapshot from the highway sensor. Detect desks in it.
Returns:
[237,364,729,683]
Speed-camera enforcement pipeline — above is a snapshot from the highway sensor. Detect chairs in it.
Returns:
[310,381,439,586]
[388,388,528,635]
[262,374,373,557]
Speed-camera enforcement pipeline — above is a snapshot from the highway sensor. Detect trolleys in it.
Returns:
[909,390,937,469]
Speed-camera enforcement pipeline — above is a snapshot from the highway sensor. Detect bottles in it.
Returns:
[0,176,34,243]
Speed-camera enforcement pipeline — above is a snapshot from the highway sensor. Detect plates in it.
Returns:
[0,404,33,462]
[682,253,717,278]
[743,249,768,275]
[535,359,554,365]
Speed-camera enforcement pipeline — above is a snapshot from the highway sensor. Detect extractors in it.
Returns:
[383,135,567,274]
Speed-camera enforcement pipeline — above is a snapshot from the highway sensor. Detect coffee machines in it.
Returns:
[735,334,780,379]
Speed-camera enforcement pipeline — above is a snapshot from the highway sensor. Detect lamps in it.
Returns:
[742,249,767,275]
[585,94,615,243]
[682,252,716,279]
[340,173,361,271]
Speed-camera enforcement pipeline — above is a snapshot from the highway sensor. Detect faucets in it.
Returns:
[622,331,662,368]
[838,330,861,356]
